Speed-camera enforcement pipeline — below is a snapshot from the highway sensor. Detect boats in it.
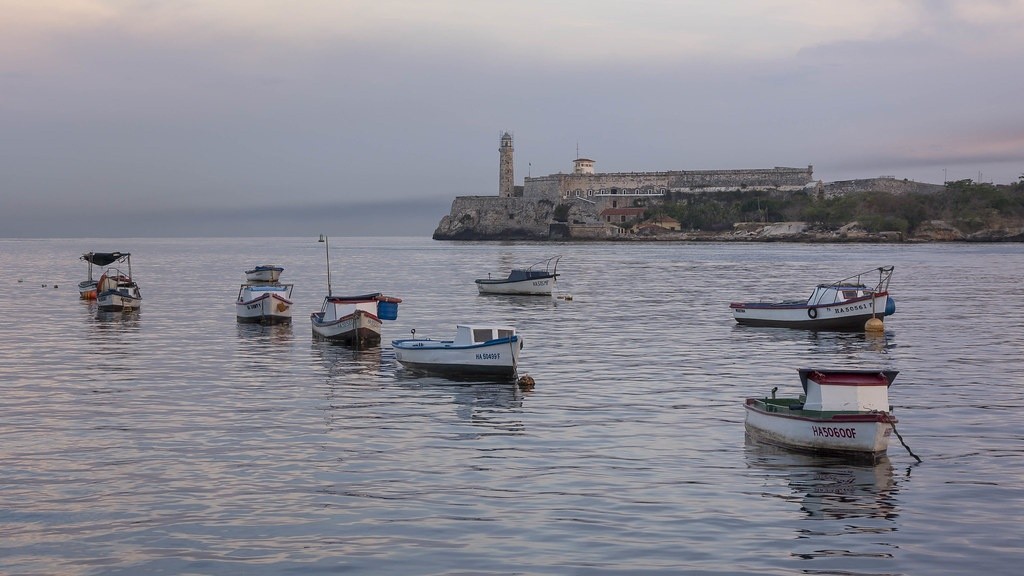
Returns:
[390,324,523,379]
[310,235,383,351]
[236,283,294,325]
[475,254,564,296]
[79,250,142,312]
[729,264,895,329]
[743,370,900,455]
[245,264,285,281]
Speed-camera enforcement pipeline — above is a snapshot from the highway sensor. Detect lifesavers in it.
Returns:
[808,307,817,318]
